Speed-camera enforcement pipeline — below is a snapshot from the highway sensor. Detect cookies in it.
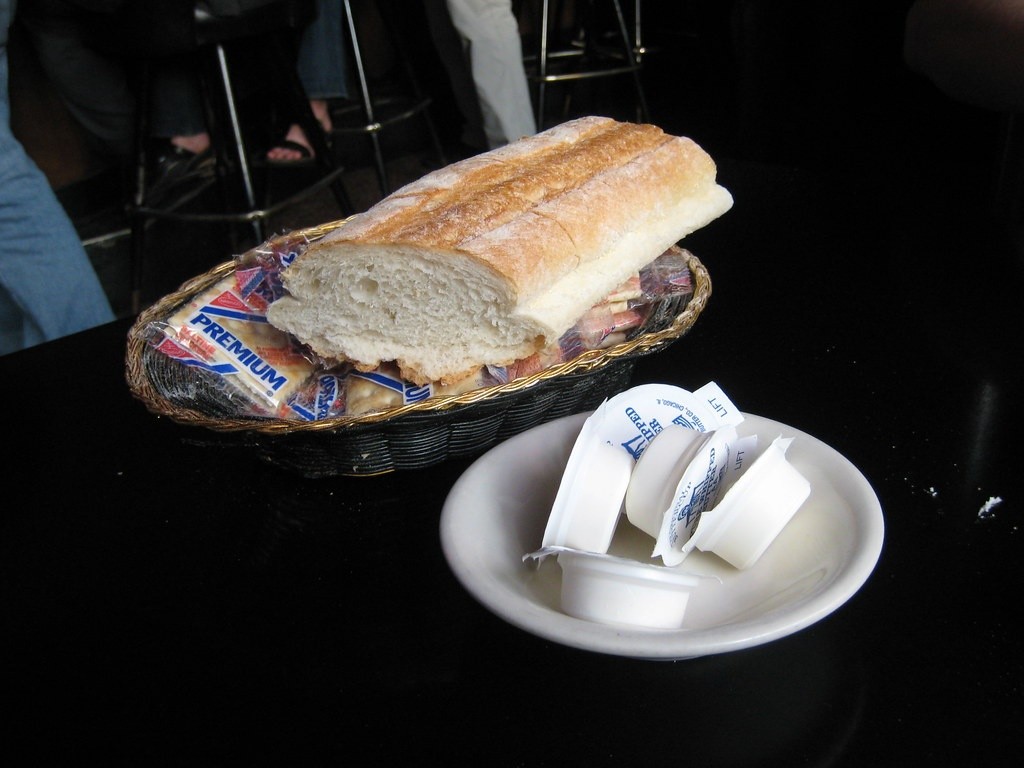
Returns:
[320,255,693,416]
[146,279,317,423]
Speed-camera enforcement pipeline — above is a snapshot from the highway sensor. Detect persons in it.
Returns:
[2,0,117,354]
[12,2,344,191]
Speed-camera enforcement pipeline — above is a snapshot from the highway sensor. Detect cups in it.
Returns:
[538,368,813,632]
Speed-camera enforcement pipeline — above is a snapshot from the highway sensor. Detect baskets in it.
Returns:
[124,214,712,478]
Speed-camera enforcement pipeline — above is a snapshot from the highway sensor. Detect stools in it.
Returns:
[126,1,649,313]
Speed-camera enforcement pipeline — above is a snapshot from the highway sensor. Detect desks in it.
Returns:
[0,139,1023,768]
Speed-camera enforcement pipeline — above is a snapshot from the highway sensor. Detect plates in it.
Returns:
[440,410,885,659]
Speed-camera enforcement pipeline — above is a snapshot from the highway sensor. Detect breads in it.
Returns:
[265,114,735,387]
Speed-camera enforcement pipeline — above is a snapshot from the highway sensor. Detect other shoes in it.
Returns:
[145,141,217,200]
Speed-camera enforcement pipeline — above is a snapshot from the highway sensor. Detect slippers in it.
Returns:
[266,117,337,169]
